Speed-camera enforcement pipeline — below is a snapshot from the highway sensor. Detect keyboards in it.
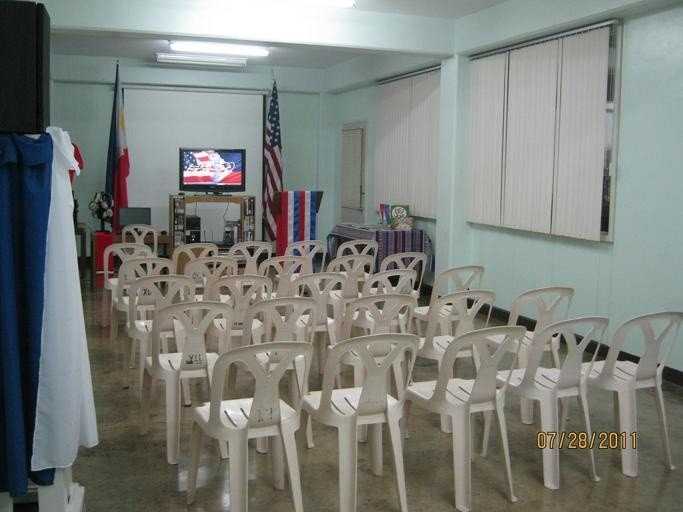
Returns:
[116,231,153,235]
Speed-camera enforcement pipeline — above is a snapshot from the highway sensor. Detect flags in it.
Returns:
[263,81,284,242]
[105,64,130,232]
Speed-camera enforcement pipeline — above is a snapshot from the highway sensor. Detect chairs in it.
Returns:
[96,221,682,512]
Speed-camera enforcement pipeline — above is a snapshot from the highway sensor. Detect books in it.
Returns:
[242,195,254,241]
[174,195,184,248]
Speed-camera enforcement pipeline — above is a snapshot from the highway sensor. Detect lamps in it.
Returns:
[154,51,248,68]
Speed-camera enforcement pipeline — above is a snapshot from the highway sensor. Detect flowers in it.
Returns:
[88,188,115,221]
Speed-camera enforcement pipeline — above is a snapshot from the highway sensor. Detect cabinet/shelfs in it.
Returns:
[168,193,255,265]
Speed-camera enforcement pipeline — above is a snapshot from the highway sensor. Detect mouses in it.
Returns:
[161,231,167,235]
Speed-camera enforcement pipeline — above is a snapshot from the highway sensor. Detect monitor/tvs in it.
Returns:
[119,208,151,231]
[179,147,246,196]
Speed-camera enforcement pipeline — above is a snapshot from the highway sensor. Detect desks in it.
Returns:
[74,221,87,278]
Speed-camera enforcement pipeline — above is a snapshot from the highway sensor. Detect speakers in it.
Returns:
[186,215,200,243]
[223,231,234,246]
